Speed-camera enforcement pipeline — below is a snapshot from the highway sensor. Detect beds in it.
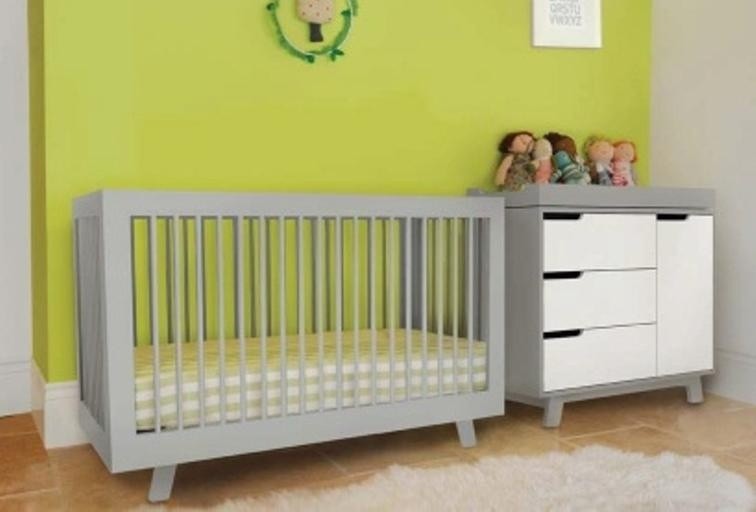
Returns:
[67,185,505,500]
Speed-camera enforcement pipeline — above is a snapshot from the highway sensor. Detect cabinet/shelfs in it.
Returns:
[463,183,717,432]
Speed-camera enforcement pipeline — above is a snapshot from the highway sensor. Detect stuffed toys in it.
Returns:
[494,131,636,191]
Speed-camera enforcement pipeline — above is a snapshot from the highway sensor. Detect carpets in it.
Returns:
[208,441,756,510]
